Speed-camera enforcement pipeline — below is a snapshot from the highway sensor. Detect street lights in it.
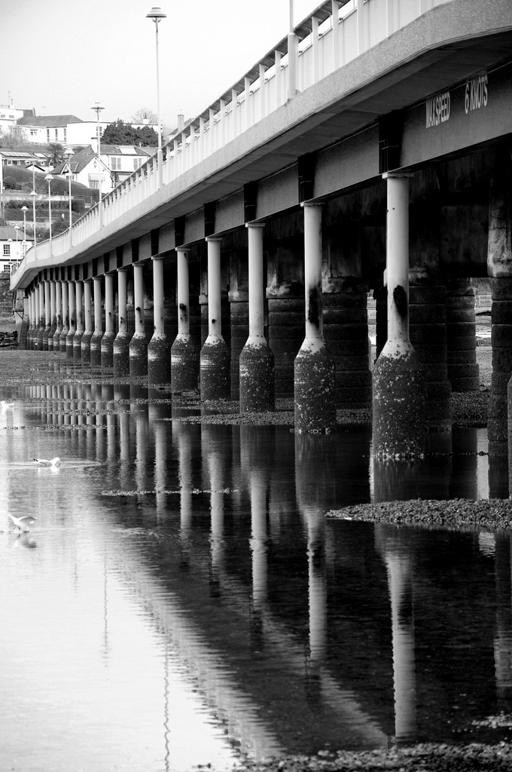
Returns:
[6,103,107,279]
[145,7,171,188]
